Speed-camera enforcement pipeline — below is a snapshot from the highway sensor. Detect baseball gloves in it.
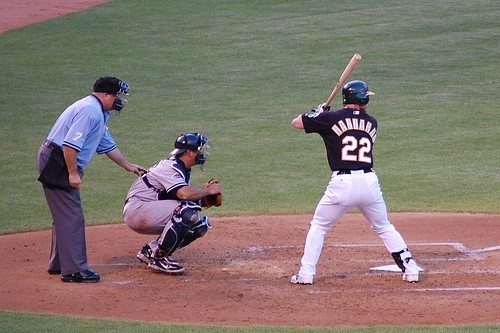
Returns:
[203,181,221,208]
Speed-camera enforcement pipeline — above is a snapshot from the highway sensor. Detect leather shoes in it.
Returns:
[48,268,60,275]
[61,268,100,283]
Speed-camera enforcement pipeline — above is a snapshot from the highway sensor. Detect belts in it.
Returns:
[43,139,55,150]
[337,169,373,175]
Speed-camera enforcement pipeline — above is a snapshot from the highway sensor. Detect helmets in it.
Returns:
[342,80,376,104]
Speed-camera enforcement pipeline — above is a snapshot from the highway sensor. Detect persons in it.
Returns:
[291,80,419,285]
[37,77,150,282]
[123,132,222,274]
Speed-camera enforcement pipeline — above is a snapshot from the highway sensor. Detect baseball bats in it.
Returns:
[324,54,362,108]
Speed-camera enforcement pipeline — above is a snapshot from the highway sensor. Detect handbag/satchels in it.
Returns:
[37,146,84,193]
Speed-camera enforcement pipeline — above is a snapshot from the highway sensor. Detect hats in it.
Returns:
[93,77,130,97]
[170,133,200,155]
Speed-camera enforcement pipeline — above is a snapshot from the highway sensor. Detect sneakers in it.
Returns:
[136,244,153,264]
[148,255,186,274]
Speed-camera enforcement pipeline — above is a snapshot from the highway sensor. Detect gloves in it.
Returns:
[315,103,331,112]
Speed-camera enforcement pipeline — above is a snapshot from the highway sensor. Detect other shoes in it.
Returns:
[402,273,419,283]
[290,275,313,285]
